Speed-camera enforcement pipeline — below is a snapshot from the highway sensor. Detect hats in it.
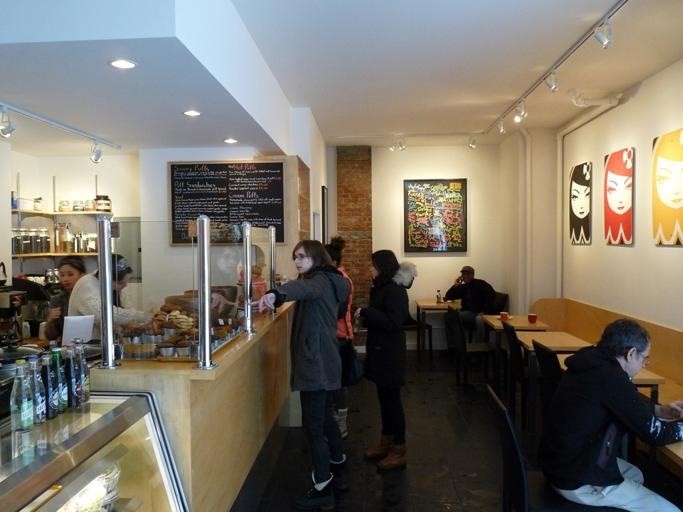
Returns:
[460,265,474,273]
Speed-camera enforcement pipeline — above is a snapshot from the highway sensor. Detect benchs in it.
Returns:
[529,296,682,483]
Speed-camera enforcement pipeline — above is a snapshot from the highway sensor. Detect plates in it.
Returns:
[496,316,512,320]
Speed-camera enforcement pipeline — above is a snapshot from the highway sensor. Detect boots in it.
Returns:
[377,445,405,468]
[333,408,348,438]
[295,471,335,511]
[328,453,348,491]
[372,437,391,454]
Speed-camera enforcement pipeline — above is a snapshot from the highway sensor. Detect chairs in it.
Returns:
[445,309,492,384]
[484,382,547,512]
[471,292,512,340]
[533,338,564,409]
[502,321,535,433]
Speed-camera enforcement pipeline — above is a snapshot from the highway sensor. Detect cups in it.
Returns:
[528,314,538,323]
[500,312,509,320]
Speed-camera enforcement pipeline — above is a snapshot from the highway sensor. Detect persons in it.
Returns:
[68,254,167,347]
[250,240,347,508]
[543,318,683,509]
[445,266,496,342]
[651,129,683,246]
[354,249,410,469]
[605,149,633,245]
[569,164,591,245]
[45,256,86,340]
[321,236,356,441]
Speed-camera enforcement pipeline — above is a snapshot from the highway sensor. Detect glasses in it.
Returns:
[636,349,650,365]
[292,254,312,259]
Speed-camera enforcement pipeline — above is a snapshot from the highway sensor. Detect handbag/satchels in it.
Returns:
[339,302,362,386]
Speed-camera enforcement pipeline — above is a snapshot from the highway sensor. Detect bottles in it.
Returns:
[436,290,441,303]
[10,339,90,434]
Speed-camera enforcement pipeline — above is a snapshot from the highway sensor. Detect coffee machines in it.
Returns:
[0,284,28,350]
